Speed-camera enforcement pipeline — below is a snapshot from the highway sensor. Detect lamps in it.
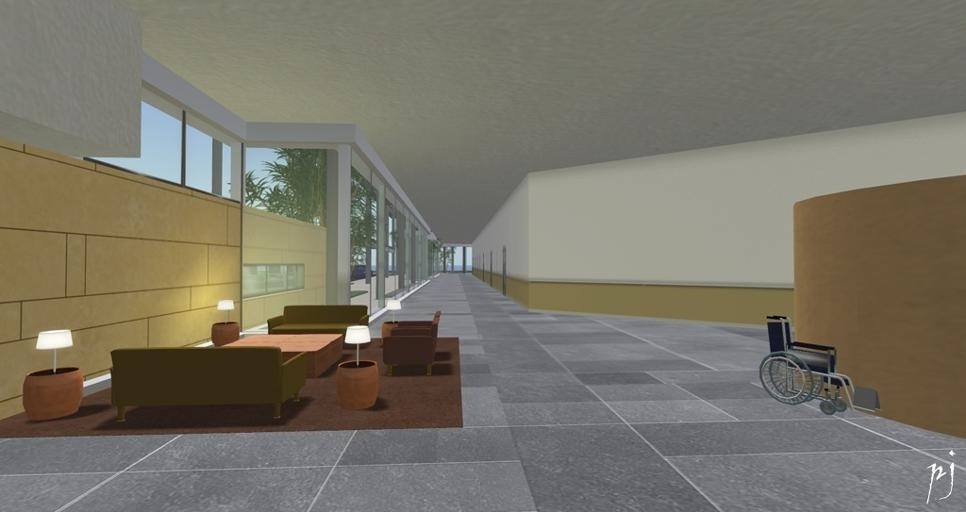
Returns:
[211,299,240,346]
[382,299,401,337]
[336,325,378,409]
[22,330,83,422]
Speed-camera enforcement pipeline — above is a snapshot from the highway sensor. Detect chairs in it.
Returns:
[382,311,440,376]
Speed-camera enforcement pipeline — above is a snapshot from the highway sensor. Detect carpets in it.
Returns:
[1,336,462,438]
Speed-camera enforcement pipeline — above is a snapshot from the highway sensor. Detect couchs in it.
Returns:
[110,305,370,422]
